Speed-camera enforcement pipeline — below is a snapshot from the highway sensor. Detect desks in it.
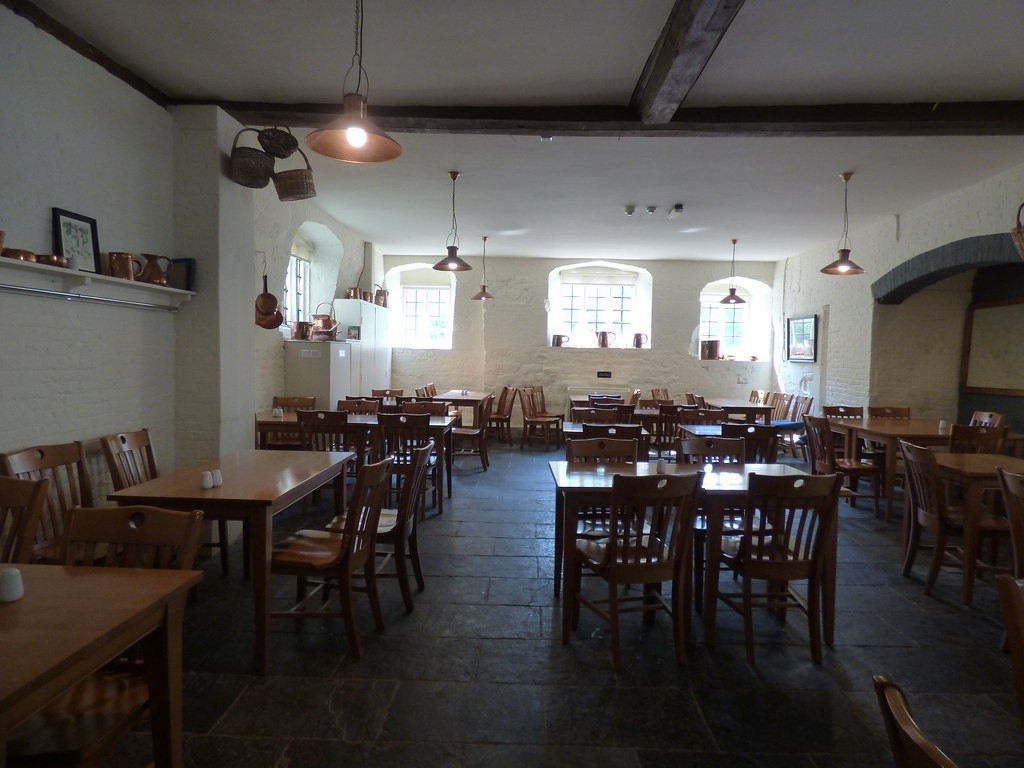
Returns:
[105,449,357,674]
[549,460,811,643]
[704,399,776,426]
[434,390,494,447]
[563,422,650,460]
[258,412,455,514]
[678,425,723,463]
[830,419,985,523]
[902,450,1024,605]
[0,564,204,768]
[569,395,590,422]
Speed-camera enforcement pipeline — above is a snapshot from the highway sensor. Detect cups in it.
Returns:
[67,257,78,270]
[377,289,388,307]
[375,296,384,307]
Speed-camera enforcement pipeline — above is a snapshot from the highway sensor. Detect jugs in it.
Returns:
[140,254,173,286]
[632,333,648,348]
[701,340,726,360]
[552,335,569,347]
[595,332,616,347]
[347,287,373,303]
[311,302,339,340]
[166,258,195,290]
[291,321,312,340]
[109,252,143,280]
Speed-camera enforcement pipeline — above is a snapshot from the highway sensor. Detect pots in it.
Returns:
[255,274,284,330]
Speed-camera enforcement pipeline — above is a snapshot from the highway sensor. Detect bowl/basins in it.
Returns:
[312,330,333,341]
[35,255,67,268]
[1,249,36,262]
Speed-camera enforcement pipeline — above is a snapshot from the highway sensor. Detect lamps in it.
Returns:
[470,235,493,300]
[302,0,403,162]
[721,241,745,303]
[434,170,472,271]
[820,172,866,276]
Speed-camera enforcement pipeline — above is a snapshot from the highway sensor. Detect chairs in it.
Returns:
[0,384,1024,768]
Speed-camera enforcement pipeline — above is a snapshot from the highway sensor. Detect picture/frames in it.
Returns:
[786,314,816,363]
[49,208,102,275]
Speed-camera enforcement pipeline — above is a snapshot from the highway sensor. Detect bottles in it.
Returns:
[461,390,467,395]
[939,420,948,429]
[657,458,665,474]
[272,408,283,417]
[0,567,24,601]
[594,392,598,395]
[200,471,213,488]
[212,469,222,486]
